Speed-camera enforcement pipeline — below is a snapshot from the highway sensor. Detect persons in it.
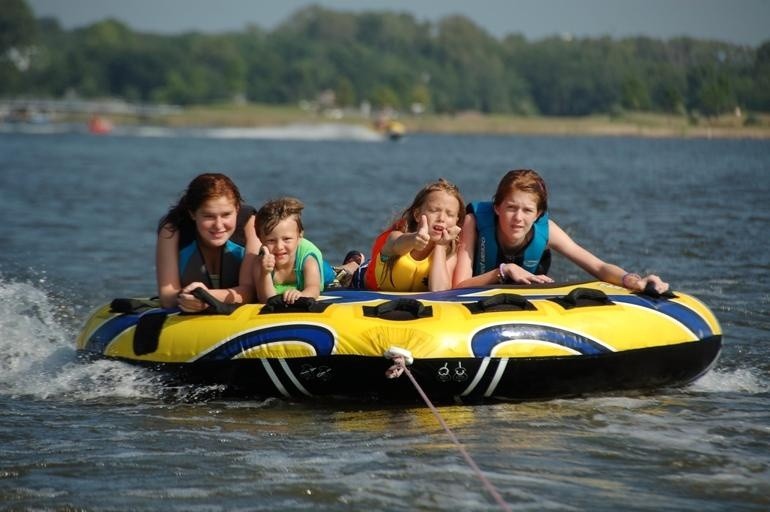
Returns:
[453,169,671,295]
[158,172,262,315]
[351,178,467,291]
[253,197,364,303]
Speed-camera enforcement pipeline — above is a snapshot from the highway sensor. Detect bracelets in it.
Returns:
[498,262,509,284]
[622,272,641,288]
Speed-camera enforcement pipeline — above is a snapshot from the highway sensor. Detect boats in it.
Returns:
[77,279,725,406]
